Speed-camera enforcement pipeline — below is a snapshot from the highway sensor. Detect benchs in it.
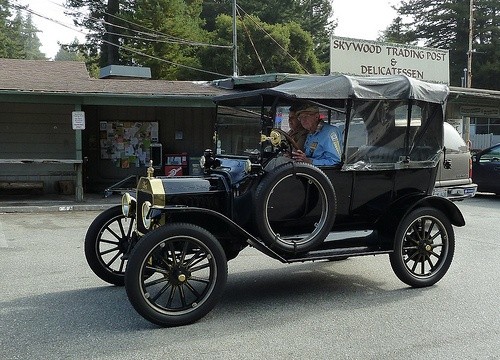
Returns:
[347,146,434,162]
[0,180,44,196]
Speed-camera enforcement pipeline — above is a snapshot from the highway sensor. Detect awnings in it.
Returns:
[208,72,500,109]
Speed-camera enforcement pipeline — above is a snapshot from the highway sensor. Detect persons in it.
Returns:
[295,105,344,169]
[272,102,309,161]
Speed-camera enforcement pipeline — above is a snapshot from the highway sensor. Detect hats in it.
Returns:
[295,103,320,118]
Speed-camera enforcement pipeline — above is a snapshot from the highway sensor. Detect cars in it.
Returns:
[82,73,466,327]
[471,142,500,198]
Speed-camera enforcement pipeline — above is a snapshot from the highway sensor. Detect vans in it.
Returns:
[318,117,478,215]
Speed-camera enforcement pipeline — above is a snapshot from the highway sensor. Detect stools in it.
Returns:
[58,180,74,195]
